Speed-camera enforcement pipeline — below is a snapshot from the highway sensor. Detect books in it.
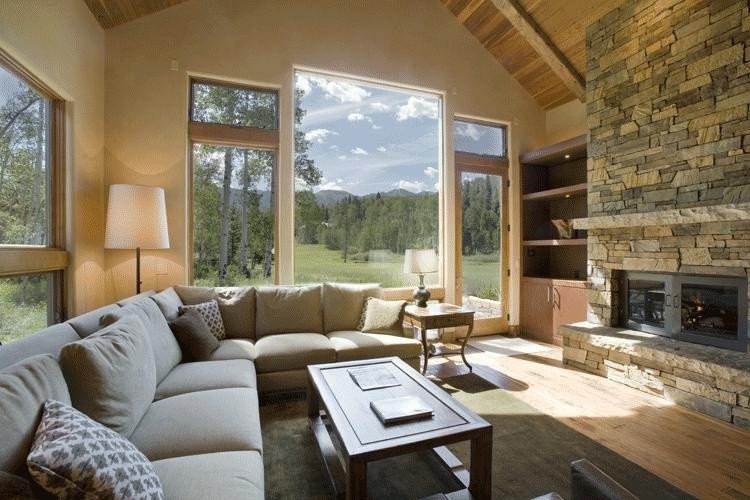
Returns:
[370,394,436,425]
[347,365,402,392]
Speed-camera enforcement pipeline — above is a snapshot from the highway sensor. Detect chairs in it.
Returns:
[528,454,641,500]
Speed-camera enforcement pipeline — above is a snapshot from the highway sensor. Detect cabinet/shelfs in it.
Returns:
[517,132,587,349]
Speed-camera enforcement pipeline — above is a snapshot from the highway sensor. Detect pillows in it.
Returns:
[180,297,228,342]
[356,294,410,337]
[166,308,221,363]
[24,395,166,500]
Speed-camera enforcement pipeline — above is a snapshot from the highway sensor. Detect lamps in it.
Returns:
[104,183,171,294]
[402,247,441,309]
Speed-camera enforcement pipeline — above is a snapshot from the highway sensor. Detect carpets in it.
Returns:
[258,371,701,500]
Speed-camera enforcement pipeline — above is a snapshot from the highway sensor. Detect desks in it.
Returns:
[402,301,476,377]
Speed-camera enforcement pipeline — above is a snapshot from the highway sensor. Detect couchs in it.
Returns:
[1,289,266,500]
[172,281,423,407]
